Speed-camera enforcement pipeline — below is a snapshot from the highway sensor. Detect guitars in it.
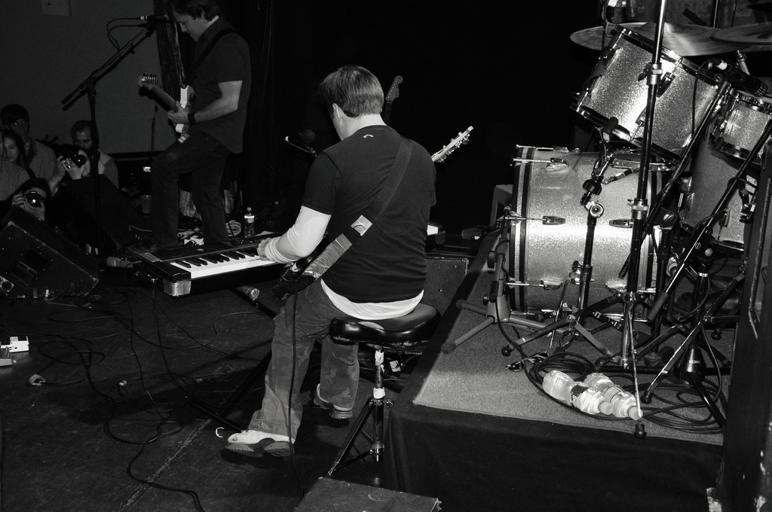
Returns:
[137,73,198,134]
[271,126,475,304]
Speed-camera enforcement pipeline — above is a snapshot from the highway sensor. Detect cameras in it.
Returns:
[61,155,86,169]
[23,191,41,208]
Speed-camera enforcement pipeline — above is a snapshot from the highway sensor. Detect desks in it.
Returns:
[387,224,725,510]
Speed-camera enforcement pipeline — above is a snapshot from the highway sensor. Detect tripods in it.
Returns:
[502,94,772,439]
[441,205,570,354]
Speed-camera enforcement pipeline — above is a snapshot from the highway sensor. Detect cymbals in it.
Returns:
[712,23,771,45]
[571,21,749,57]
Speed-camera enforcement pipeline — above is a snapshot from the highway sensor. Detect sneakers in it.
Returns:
[313,384,353,419]
[225,429,296,458]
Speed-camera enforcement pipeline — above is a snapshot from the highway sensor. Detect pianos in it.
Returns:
[142,233,283,296]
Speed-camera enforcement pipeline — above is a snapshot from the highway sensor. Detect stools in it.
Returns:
[325,304,440,489]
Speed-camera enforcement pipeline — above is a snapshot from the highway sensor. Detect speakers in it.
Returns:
[719,175,772,512]
[0,205,100,299]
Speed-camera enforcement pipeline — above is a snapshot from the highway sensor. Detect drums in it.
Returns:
[709,88,772,164]
[575,26,722,165]
[677,125,761,252]
[508,144,664,312]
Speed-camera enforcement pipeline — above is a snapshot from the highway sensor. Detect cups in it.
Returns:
[141,194,153,215]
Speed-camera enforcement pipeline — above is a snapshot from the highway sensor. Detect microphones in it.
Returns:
[139,12,174,22]
[710,55,768,98]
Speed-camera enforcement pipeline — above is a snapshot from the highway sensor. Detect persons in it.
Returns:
[0,105,119,294]
[223,66,436,457]
[609,2,756,29]
[151,1,252,248]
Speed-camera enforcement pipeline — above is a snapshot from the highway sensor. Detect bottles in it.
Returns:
[244,206,255,238]
[582,372,643,421]
[541,369,614,415]
